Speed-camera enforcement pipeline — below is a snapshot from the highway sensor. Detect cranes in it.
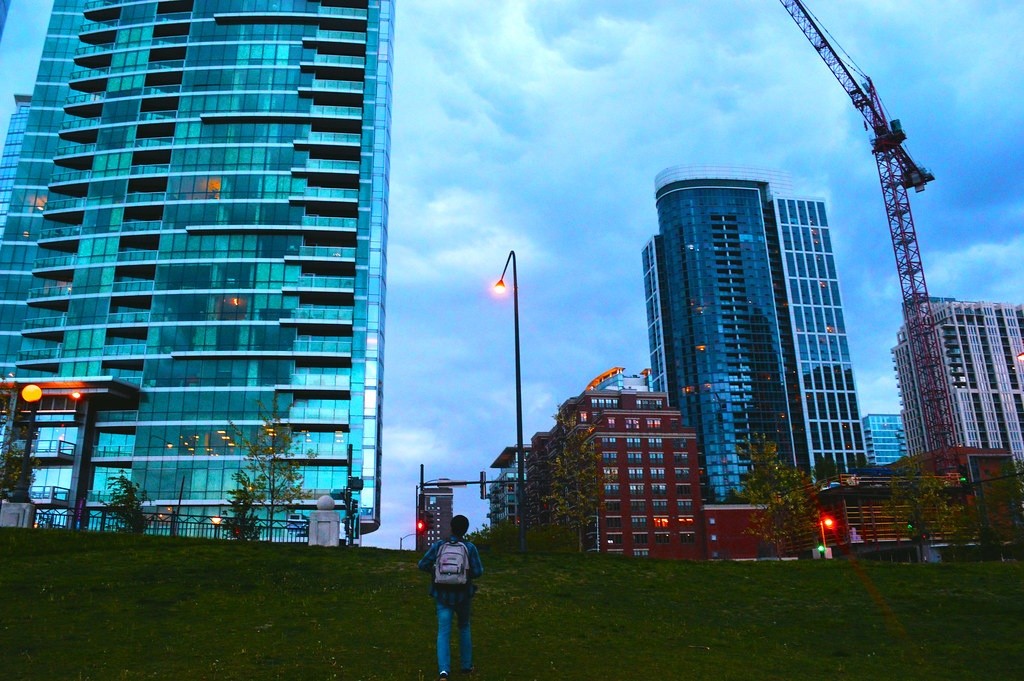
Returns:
[780,0,966,478]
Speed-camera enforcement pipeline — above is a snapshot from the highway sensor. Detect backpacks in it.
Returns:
[434,536,469,585]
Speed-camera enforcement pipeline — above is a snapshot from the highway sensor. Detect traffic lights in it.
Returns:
[960,473,969,488]
[417,519,424,532]
[921,530,930,539]
[907,521,915,538]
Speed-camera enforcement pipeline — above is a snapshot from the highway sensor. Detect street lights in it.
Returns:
[9,386,42,501]
[415,477,450,551]
[494,250,527,550]
[820,516,833,549]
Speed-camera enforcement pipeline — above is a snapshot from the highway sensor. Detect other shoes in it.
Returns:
[439,673,448,681]
[461,666,475,675]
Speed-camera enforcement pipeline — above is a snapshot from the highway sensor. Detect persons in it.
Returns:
[418,515,483,681]
